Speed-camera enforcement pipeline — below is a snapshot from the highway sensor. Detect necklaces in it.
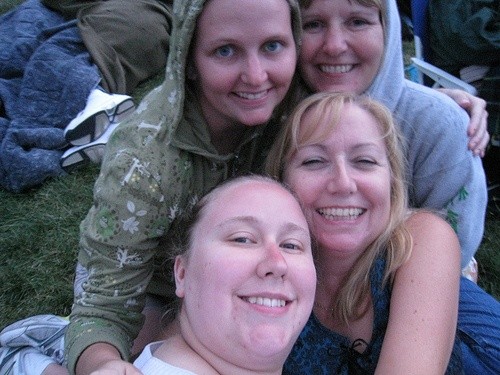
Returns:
[314,298,338,312]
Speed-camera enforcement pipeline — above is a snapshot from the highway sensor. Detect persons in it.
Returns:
[294,1,488,271]
[27,0,171,168]
[9,91,500,375]
[63,0,488,375]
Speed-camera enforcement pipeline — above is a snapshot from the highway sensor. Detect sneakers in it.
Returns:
[0,314,69,364]
[0,346,53,375]
[64,89,136,146]
[60,123,125,174]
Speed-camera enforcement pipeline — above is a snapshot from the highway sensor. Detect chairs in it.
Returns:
[410,0,477,97]
